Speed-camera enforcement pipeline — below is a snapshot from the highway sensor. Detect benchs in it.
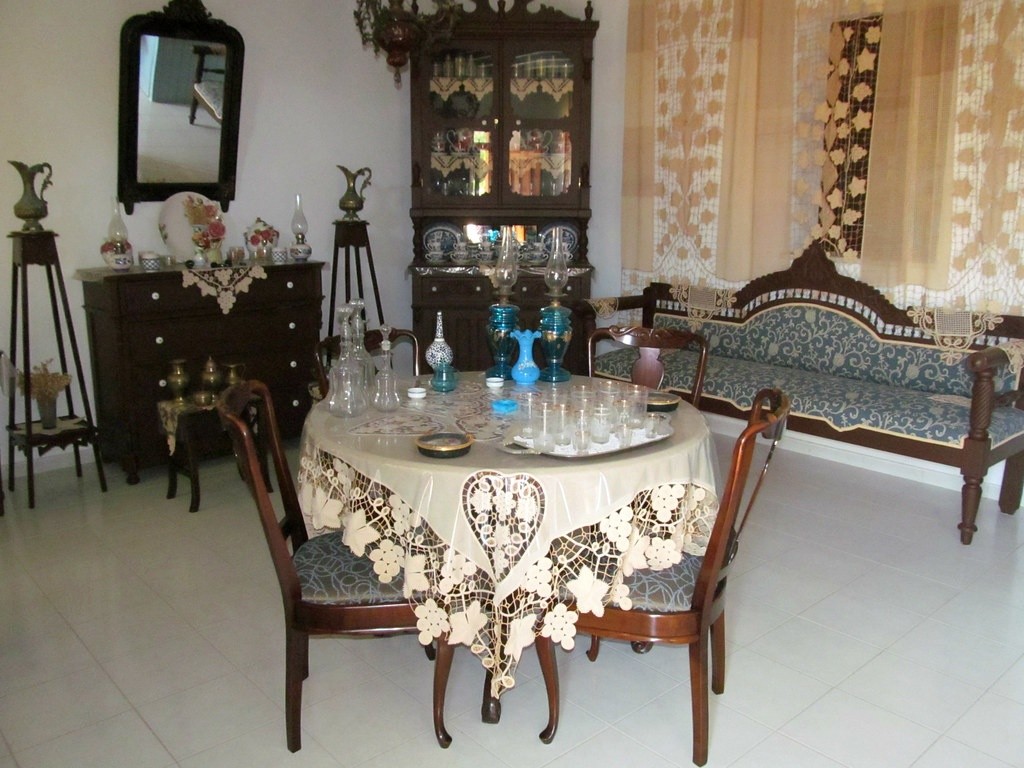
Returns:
[571,237,1024,546]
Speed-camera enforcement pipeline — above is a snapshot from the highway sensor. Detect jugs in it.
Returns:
[431,361,460,391]
[337,165,372,222]
[224,363,246,391]
[522,128,553,153]
[7,160,53,231]
[430,128,473,155]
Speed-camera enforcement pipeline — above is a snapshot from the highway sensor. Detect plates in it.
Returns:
[422,222,463,260]
[159,192,226,259]
[538,221,580,260]
[647,391,680,411]
[415,433,473,457]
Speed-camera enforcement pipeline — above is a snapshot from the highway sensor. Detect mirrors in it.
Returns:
[116,1,246,215]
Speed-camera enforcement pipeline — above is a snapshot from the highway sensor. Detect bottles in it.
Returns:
[328,304,367,417]
[372,324,402,412]
[350,298,375,399]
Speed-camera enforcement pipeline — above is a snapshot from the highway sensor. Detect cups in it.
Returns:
[450,252,468,261]
[272,248,287,264]
[230,247,244,266]
[160,255,176,271]
[142,253,160,273]
[513,252,523,262]
[563,252,573,261]
[425,252,443,260]
[249,252,260,264]
[476,251,493,261]
[193,391,212,406]
[453,242,467,252]
[531,252,548,263]
[478,241,492,252]
[517,381,670,454]
[427,242,441,252]
[512,245,523,253]
[562,242,571,251]
[533,242,545,252]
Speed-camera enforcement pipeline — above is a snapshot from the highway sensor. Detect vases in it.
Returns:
[37,399,58,429]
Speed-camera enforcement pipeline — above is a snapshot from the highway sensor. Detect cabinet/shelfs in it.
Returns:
[77,251,327,485]
[6,230,108,511]
[326,221,385,338]
[408,0,599,381]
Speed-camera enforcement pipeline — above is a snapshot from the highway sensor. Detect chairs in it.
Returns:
[525,387,791,767]
[585,324,709,411]
[313,326,422,398]
[216,379,472,753]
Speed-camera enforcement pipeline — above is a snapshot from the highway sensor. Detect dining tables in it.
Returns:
[299,368,723,724]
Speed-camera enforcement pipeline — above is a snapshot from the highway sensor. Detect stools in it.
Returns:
[156,391,275,514]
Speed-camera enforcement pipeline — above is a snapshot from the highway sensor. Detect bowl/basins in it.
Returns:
[486,378,504,388]
[408,388,427,399]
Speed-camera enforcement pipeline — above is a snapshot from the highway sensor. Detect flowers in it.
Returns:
[13,359,73,400]
